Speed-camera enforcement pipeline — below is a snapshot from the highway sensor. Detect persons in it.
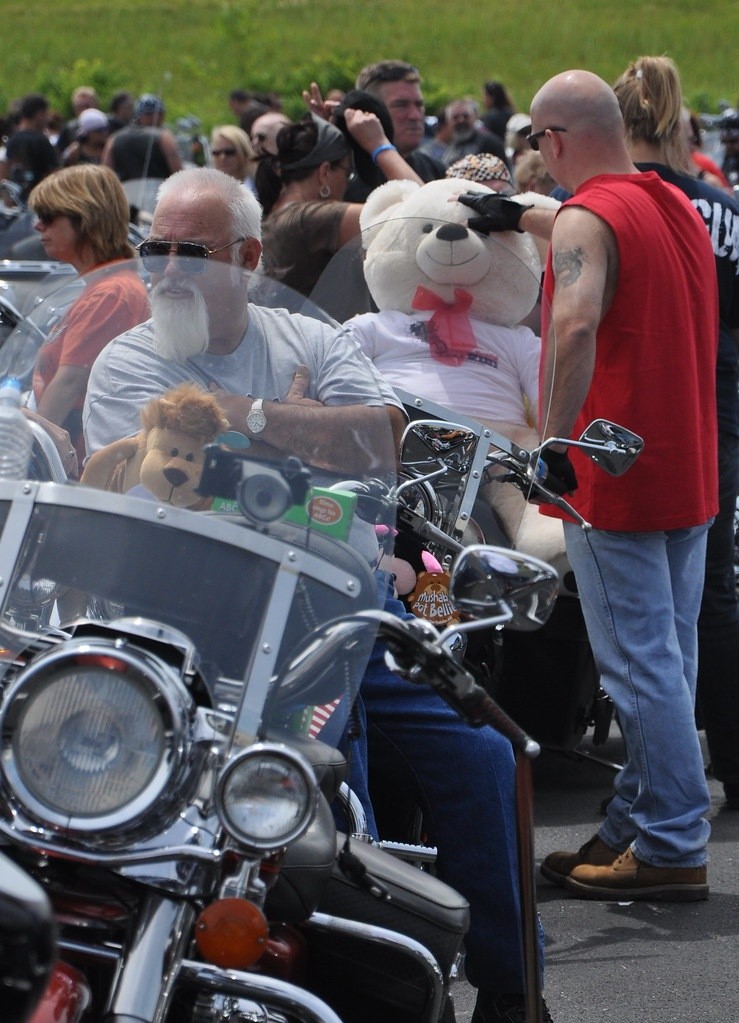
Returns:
[458,49,739,897]
[210,58,522,323]
[82,167,553,1023]
[1,85,211,475]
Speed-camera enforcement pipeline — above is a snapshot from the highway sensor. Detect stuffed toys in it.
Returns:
[126,382,232,511]
[341,177,572,580]
[373,522,461,633]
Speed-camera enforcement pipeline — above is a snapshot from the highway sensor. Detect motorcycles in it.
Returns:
[0,256,645,1022]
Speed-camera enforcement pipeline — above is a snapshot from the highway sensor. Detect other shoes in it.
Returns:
[469,988,553,1023]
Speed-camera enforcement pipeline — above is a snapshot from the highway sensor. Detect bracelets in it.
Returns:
[372,144,396,163]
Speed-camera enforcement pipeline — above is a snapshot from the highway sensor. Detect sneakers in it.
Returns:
[540,835,622,884]
[565,844,709,900]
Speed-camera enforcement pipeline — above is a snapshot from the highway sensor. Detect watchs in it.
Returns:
[246,397,266,444]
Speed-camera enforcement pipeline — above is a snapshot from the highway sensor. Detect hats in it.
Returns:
[73,108,110,135]
[445,153,514,188]
[506,113,532,133]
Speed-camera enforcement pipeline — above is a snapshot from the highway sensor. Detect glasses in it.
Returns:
[134,237,244,274]
[39,210,66,227]
[363,65,419,91]
[250,134,266,142]
[527,126,566,150]
[212,149,236,158]
